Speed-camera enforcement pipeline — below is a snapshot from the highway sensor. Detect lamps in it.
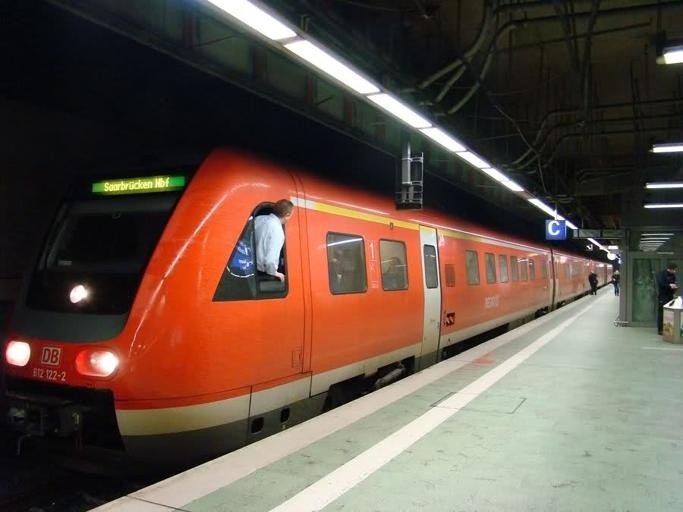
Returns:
[640,41,682,211]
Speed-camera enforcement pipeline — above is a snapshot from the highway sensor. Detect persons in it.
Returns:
[589,270,598,295]
[655,262,680,335]
[613,269,620,296]
[255,198,294,285]
[332,247,361,289]
[384,257,403,289]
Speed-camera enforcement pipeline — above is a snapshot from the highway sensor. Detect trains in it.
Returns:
[0,141,613,485]
